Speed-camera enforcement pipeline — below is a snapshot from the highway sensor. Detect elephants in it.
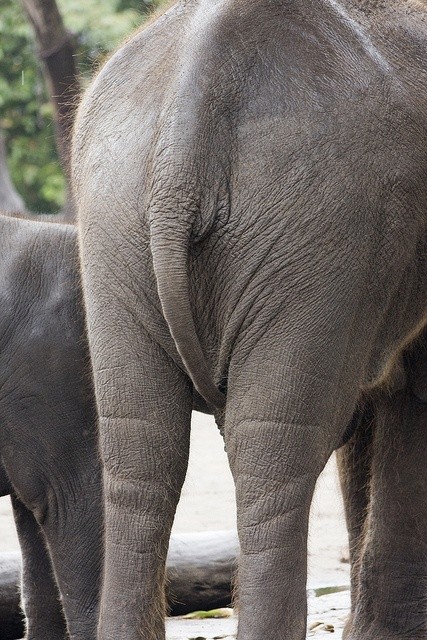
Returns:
[0,217,102,640]
[69,1,426,639]
